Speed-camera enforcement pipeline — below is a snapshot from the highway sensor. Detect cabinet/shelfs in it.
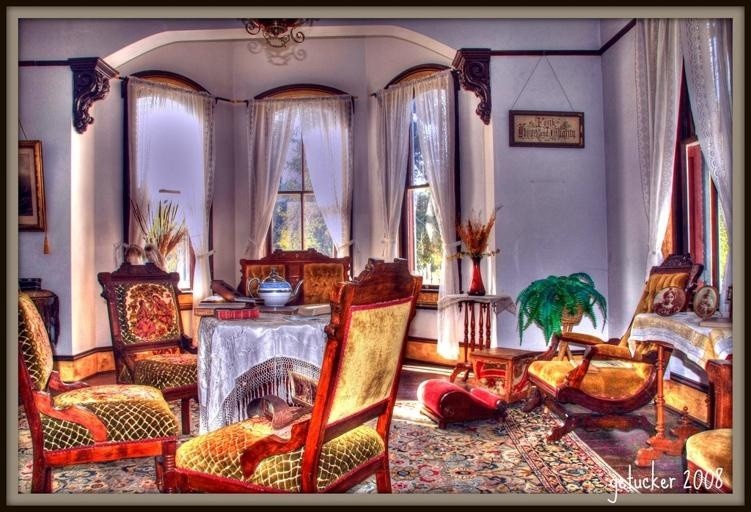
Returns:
[443,294,512,383]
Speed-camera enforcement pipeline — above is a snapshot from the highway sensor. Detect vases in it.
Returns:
[466,255,487,296]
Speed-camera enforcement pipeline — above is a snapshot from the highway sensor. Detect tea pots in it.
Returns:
[247,268,305,308]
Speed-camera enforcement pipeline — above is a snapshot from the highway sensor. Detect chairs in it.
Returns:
[685,353,733,494]
[18,247,424,494]
[520,252,704,444]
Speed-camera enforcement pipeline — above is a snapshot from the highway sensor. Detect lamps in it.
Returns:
[233,18,319,49]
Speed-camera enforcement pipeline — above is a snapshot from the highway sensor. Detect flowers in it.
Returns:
[444,207,501,260]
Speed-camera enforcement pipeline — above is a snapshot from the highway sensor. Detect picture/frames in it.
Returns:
[18,139,47,232]
[692,285,720,317]
[509,109,585,149]
[651,286,687,317]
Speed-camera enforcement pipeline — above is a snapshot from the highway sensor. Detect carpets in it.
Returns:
[19,394,642,493]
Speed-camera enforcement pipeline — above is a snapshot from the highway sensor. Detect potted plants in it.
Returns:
[514,272,611,346]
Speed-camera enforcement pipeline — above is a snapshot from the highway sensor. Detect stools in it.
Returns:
[469,347,541,405]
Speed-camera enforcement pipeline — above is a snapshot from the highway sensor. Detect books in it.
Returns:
[195,278,265,320]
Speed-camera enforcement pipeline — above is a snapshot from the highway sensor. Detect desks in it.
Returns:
[632,312,733,468]
